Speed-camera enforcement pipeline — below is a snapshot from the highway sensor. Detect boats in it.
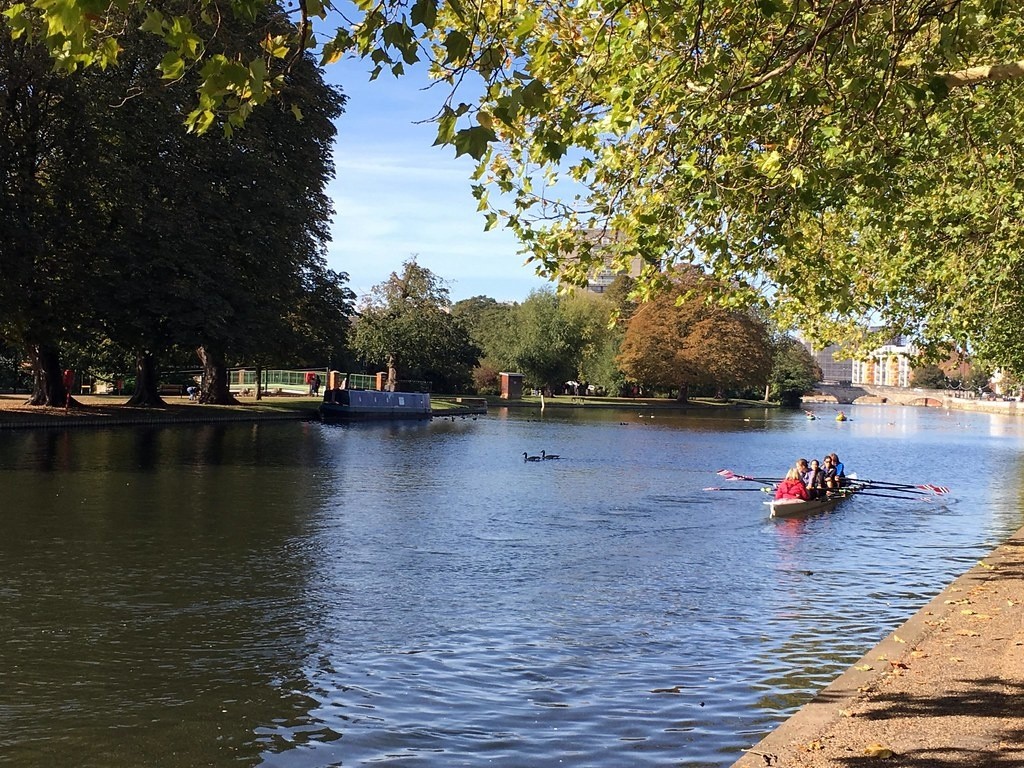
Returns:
[770,472,858,517]
[319,389,433,422]
[836,414,846,421]
[807,416,815,420]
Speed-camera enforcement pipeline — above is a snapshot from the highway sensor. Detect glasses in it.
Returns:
[825,461,831,463]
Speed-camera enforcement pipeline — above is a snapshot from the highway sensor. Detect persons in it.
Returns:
[535,385,539,395]
[187,386,200,400]
[775,452,847,501]
[310,375,321,397]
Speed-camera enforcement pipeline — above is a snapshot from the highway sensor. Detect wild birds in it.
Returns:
[618,411,752,427]
[417,411,542,423]
[521,449,561,461]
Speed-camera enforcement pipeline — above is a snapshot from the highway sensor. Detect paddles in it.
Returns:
[701,468,786,494]
[813,477,949,502]
